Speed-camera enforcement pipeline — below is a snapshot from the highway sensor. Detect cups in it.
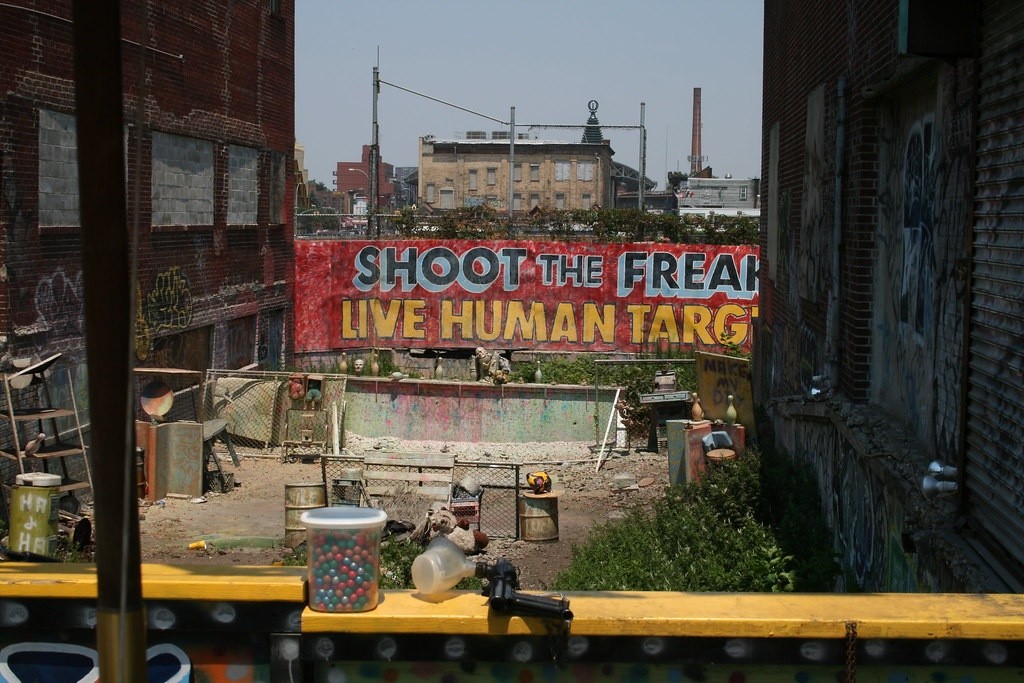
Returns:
[300,506,387,614]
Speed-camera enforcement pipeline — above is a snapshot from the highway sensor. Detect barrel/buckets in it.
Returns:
[284,482,326,548]
[517,490,560,545]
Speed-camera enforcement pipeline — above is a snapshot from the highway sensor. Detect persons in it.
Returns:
[475,346,509,378]
[353,359,364,376]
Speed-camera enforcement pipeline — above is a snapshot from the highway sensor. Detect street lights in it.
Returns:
[404,188,416,204]
[348,168,368,178]
[294,183,302,237]
